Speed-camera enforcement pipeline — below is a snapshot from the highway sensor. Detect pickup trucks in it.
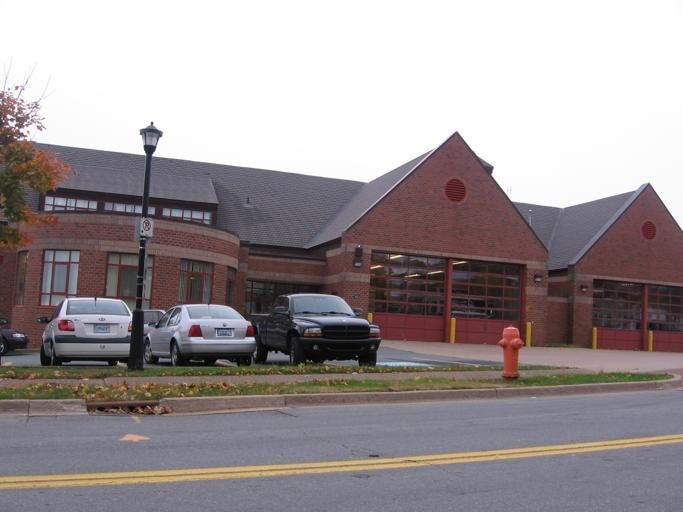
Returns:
[248,292,381,368]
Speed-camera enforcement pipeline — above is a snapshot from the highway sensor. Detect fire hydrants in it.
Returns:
[496,324,525,379]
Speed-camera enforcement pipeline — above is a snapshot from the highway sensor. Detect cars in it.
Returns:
[34,297,135,366]
[130,309,169,344]
[0,328,27,354]
[141,302,256,367]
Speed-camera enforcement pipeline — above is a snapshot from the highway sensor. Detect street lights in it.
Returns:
[127,120,164,367]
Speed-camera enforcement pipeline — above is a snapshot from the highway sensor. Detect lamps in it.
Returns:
[580,286,588,292]
[535,276,542,282]
[352,246,363,269]
